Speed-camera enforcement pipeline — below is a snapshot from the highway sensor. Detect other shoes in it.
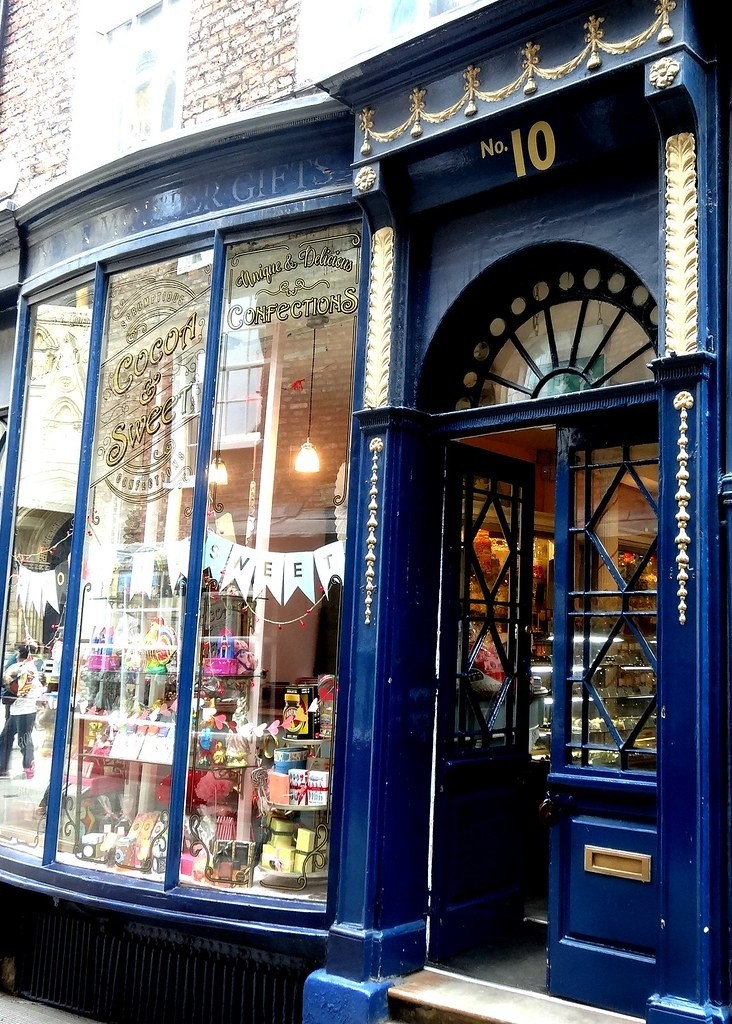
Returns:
[13,771,34,779]
[0,772,10,779]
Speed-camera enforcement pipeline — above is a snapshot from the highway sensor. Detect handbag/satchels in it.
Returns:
[2,675,19,705]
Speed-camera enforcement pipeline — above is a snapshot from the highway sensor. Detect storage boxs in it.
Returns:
[288,755,330,807]
[127,811,257,889]
[202,656,238,676]
[261,816,330,875]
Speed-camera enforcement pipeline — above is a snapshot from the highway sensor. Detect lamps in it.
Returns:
[205,244,229,486]
[296,311,328,474]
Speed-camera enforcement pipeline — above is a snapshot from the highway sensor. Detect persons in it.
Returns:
[0,638,43,778]
